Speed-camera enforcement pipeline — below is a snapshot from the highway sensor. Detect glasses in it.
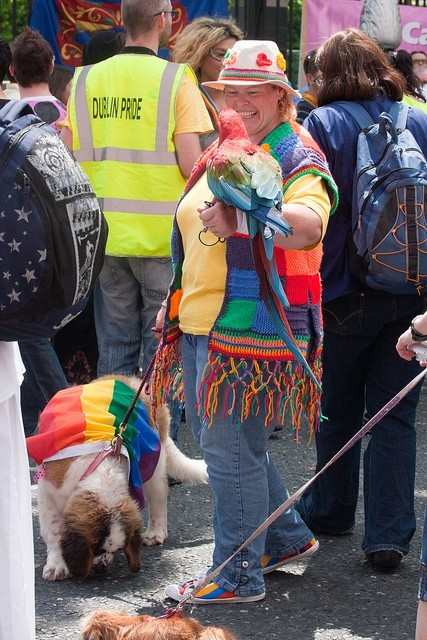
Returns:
[314,73,324,88]
[154,10,177,17]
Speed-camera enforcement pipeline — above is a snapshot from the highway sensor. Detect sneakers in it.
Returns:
[261,537,319,578]
[371,549,402,573]
[166,577,266,605]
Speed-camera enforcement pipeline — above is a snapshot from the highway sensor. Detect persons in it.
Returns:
[388,50,427,116]
[48,67,74,106]
[169,15,248,151]
[9,25,67,133]
[410,51,427,103]
[81,32,127,66]
[151,40,338,604]
[294,28,427,574]
[295,50,323,125]
[396,311,427,639]
[55,0,214,486]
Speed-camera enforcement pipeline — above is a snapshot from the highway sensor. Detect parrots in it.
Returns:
[197,97,338,395]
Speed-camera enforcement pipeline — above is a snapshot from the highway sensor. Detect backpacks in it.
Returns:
[1,98,108,342]
[333,101,427,295]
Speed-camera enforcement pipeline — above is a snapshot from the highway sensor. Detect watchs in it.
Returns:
[411,315,427,342]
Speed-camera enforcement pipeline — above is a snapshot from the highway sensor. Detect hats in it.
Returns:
[201,40,300,100]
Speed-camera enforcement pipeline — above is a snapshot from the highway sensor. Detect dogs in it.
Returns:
[35,375,210,586]
[81,608,234,640]
